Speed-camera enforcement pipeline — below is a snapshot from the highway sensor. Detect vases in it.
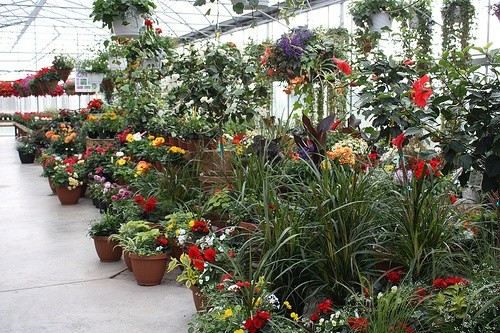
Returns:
[193,140,204,150]
[38,81,48,93]
[205,138,218,150]
[58,68,71,83]
[55,183,82,205]
[168,136,178,146]
[44,80,58,94]
[200,171,234,195]
[113,14,145,39]
[18,148,35,163]
[92,235,123,262]
[191,286,210,314]
[128,251,168,286]
[187,142,196,152]
[179,140,187,150]
[239,220,261,241]
[201,149,234,174]
[103,80,114,90]
[49,176,57,194]
[205,211,230,227]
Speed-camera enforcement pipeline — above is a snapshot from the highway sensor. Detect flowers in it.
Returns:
[0,0,500,333]
